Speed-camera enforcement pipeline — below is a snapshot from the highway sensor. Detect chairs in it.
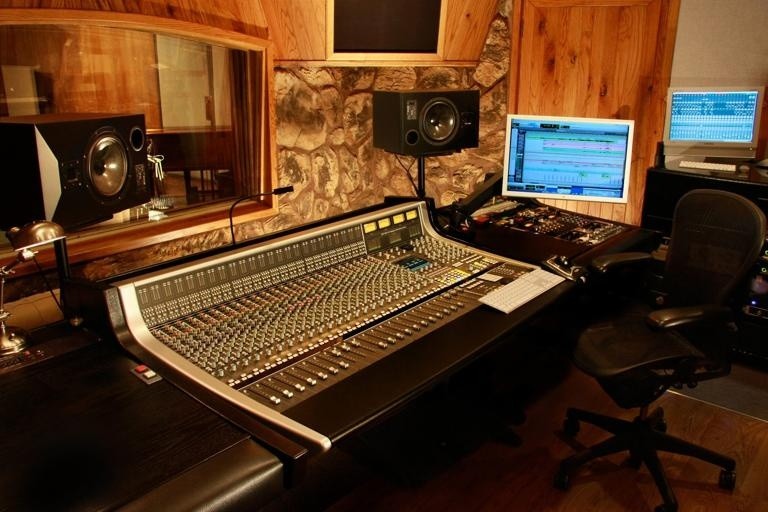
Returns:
[553,188,767,512]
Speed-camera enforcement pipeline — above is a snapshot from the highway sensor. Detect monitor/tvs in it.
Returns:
[501,113,636,203]
[662,83,767,161]
[325,0,448,70]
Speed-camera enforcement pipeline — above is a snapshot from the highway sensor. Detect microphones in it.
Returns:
[228,186,294,243]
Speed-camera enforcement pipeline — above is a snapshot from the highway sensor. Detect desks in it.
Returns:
[641,156,768,232]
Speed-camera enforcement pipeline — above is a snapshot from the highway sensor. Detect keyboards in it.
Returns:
[680,159,736,173]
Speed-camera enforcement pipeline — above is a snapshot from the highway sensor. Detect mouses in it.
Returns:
[738,164,750,170]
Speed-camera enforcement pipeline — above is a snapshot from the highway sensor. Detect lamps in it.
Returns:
[0,220,68,356]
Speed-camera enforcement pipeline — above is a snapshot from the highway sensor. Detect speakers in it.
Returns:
[371,86,481,158]
[0,113,157,233]
